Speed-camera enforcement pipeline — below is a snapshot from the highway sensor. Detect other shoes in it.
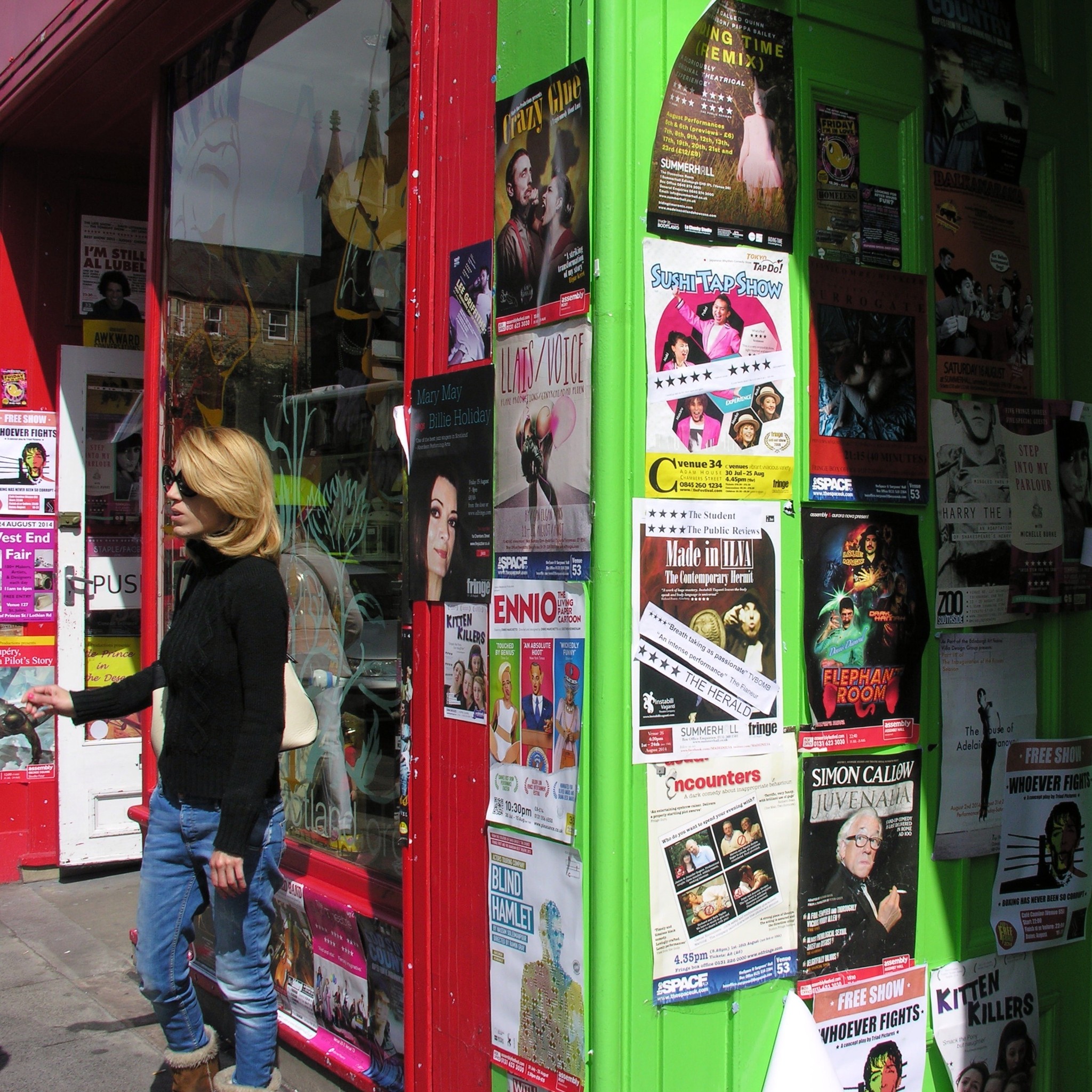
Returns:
[329,833,361,851]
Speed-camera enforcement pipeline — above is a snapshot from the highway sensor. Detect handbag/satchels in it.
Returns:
[151,653,319,763]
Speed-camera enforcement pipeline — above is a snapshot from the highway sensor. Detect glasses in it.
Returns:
[846,835,883,850]
[161,464,198,496]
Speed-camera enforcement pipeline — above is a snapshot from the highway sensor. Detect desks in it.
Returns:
[346,621,399,817]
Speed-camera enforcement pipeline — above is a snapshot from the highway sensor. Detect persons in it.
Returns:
[314,147,583,1092]
[23,424,294,1092]
[276,509,365,841]
[643,30,1092,1092]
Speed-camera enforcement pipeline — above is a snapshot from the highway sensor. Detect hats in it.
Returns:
[733,414,759,432]
[756,386,780,407]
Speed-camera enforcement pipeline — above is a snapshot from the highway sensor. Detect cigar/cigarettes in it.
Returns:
[888,889,908,893]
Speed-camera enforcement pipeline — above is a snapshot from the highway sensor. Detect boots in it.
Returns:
[163,1025,219,1092]
[214,1064,280,1092]
